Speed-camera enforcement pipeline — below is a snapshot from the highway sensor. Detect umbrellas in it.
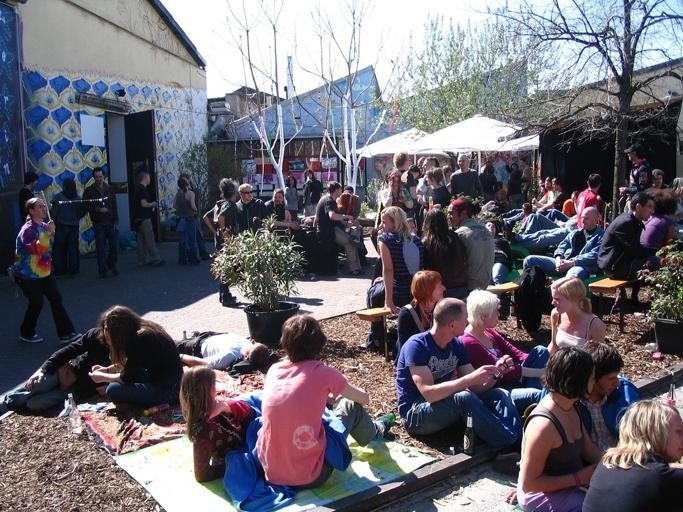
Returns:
[354,115,540,174]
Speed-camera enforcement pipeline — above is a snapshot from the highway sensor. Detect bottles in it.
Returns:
[462,408,475,456]
[374,410,397,424]
[142,402,170,417]
[345,218,352,233]
[482,362,508,391]
[428,197,433,211]
[66,392,85,435]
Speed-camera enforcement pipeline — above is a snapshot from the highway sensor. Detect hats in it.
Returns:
[624,143,643,154]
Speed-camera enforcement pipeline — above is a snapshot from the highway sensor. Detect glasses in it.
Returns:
[241,191,252,194]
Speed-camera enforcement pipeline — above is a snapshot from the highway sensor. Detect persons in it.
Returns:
[235,169,369,275]
[131,171,171,267]
[395,271,607,474]
[203,177,241,308]
[0,305,184,419]
[82,167,120,278]
[516,340,642,512]
[173,171,211,268]
[18,171,39,222]
[174,315,394,497]
[10,197,83,346]
[51,178,92,273]
[581,400,682,511]
[357,144,680,351]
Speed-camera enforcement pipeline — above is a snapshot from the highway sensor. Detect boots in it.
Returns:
[222,293,241,307]
[220,292,237,302]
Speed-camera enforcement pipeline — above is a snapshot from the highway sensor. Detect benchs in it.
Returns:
[356,282,521,357]
[588,278,636,332]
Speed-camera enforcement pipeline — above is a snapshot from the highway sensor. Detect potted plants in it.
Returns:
[637,244,683,354]
[210,214,308,346]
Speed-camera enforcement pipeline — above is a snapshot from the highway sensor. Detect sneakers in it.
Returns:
[155,261,167,266]
[59,333,82,344]
[496,451,521,473]
[18,333,43,343]
